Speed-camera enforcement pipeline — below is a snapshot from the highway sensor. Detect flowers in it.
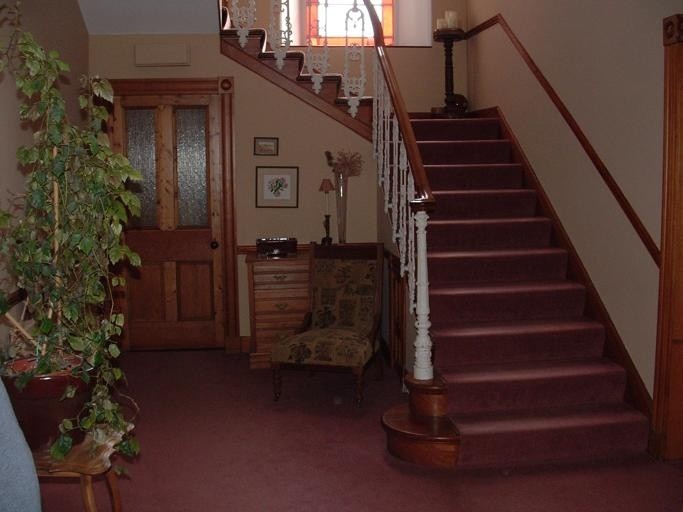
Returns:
[265,175,288,193]
[325,147,363,178]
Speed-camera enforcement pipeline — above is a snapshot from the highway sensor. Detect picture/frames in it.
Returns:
[253,136,278,156]
[255,164,298,208]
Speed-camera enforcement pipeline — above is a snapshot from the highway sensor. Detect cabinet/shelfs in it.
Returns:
[244,244,310,371]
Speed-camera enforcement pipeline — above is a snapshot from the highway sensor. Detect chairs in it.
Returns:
[269,244,381,406]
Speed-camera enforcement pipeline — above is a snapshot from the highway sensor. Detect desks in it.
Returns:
[32,419,135,511]
[431,27,465,114]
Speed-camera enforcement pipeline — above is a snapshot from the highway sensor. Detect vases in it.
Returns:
[334,172,348,246]
[273,189,280,197]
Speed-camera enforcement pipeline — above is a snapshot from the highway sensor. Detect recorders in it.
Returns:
[256,238,298,259]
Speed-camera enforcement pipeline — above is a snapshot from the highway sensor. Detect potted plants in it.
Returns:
[0,4,150,481]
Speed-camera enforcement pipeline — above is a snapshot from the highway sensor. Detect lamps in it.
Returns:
[316,178,335,245]
